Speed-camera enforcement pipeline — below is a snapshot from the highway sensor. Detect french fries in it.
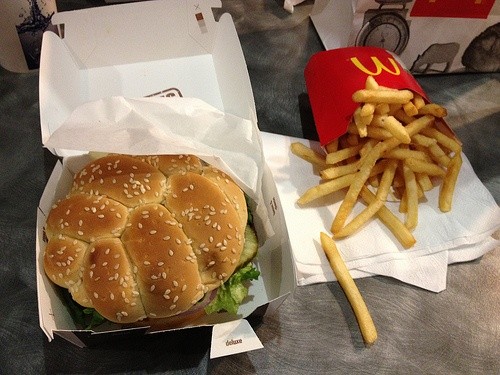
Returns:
[293,74,464,347]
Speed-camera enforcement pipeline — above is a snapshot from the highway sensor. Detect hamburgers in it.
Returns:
[45,156,262,329]
